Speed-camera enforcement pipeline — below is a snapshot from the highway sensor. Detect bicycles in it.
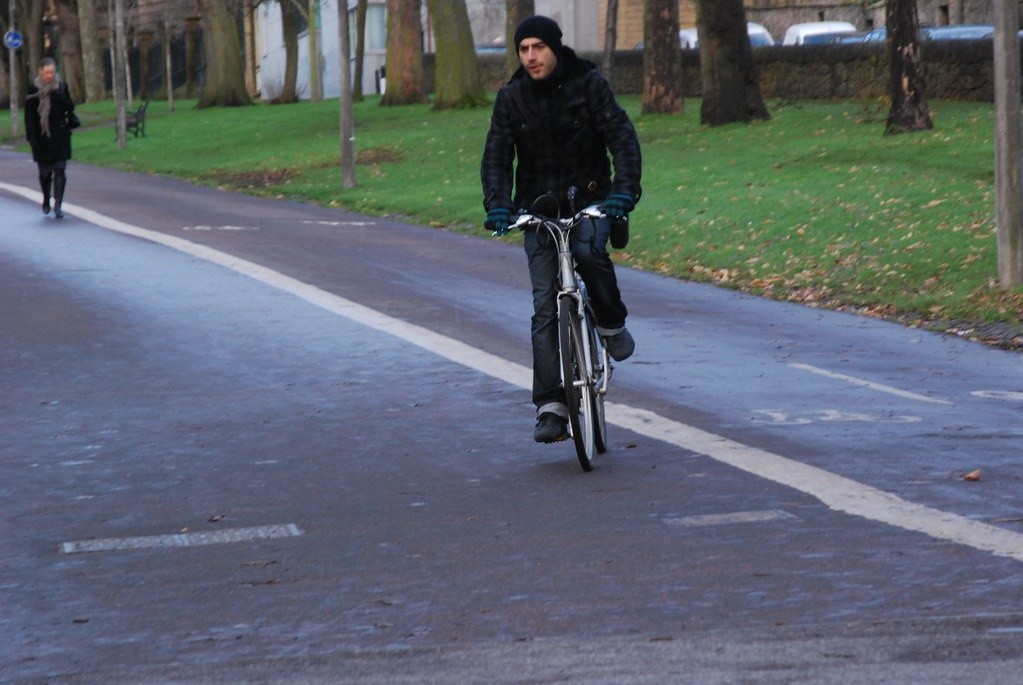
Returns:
[484,202,628,472]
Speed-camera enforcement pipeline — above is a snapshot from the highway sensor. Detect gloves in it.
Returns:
[486,207,513,237]
[29,137,40,151]
[599,194,636,218]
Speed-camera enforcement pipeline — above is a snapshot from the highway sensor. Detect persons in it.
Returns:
[480,15,642,441]
[24,57,74,218]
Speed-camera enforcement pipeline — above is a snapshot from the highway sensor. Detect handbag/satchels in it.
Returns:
[610,214,629,248]
[54,82,80,134]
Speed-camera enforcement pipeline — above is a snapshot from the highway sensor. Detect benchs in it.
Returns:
[115,100,149,142]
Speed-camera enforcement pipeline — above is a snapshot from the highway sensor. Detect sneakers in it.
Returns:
[603,328,634,361]
[534,412,566,442]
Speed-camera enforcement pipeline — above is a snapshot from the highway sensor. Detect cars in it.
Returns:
[679,21,1023,49]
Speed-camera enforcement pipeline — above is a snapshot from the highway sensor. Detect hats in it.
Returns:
[514,16,562,61]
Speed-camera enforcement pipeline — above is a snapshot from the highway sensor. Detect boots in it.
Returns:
[39,173,51,214]
[54,175,66,217]
